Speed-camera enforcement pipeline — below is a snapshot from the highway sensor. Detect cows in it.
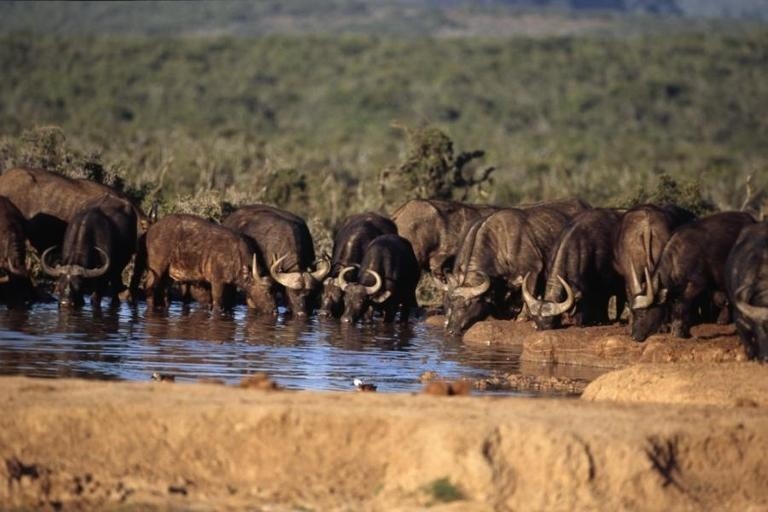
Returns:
[1,168,768,358]
